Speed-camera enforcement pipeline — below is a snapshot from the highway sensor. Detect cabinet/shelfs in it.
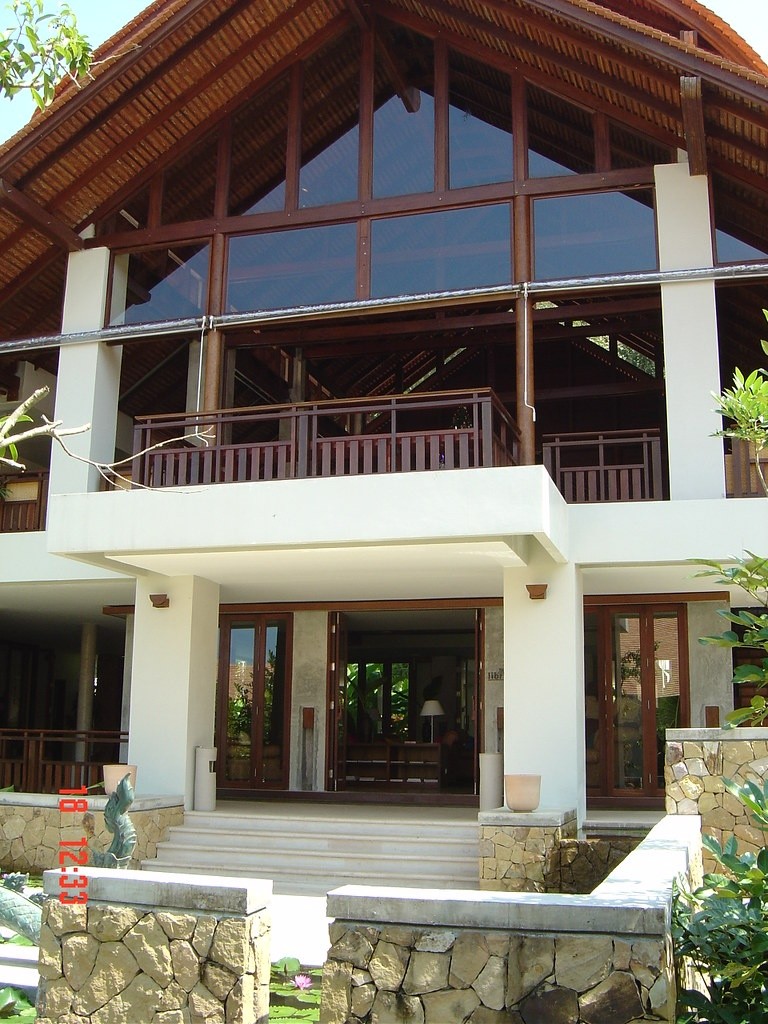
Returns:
[336,743,444,791]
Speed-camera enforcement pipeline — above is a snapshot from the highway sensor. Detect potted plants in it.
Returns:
[347,732,362,745]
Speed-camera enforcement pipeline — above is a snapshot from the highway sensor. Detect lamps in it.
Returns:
[419,700,445,743]
